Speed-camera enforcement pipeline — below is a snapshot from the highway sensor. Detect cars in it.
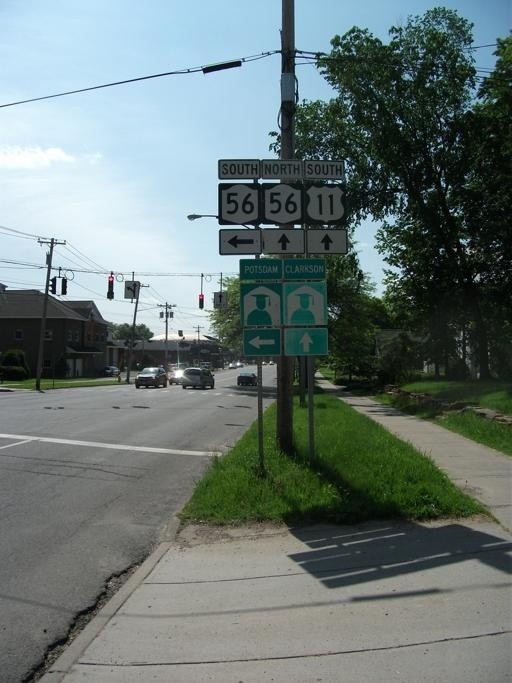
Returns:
[104,360,275,388]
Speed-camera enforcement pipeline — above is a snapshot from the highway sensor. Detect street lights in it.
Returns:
[187,212,252,228]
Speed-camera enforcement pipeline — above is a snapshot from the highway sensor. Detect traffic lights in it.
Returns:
[61,278,68,295]
[49,276,56,293]
[199,294,204,308]
[107,276,114,299]
